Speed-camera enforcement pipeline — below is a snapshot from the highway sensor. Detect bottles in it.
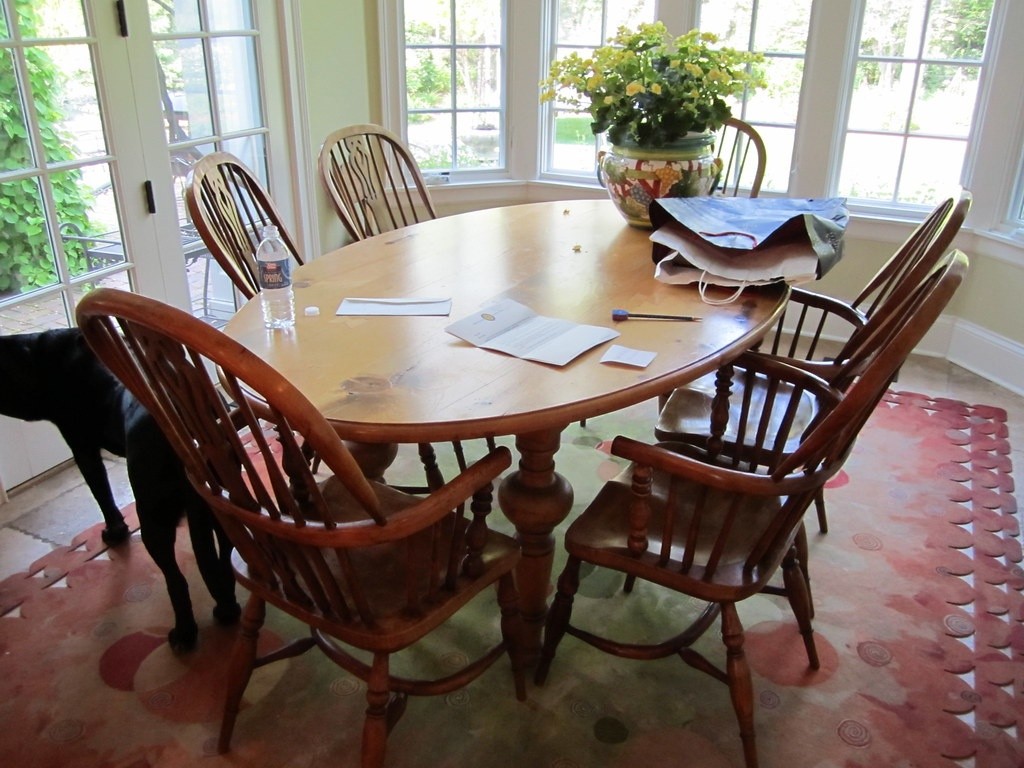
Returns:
[256,224,295,330]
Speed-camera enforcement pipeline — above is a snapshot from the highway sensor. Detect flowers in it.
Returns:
[539,16,767,140]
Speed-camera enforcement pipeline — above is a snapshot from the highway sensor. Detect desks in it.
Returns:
[213,200,791,672]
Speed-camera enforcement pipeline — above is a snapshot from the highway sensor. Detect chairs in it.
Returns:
[187,149,305,300]
[534,249,969,768]
[322,123,437,243]
[655,189,978,618]
[735,198,956,532]
[74,285,527,768]
[707,119,767,197]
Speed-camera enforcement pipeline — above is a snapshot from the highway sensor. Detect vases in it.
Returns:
[597,131,722,228]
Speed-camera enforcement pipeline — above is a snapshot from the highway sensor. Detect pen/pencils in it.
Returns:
[611,307,703,322]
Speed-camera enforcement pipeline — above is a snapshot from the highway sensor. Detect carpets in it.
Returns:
[0,388,1024,768]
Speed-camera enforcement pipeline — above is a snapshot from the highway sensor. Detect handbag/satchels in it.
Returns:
[646,197,849,305]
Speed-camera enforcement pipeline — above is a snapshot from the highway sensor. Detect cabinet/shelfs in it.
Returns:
[64,220,271,331]
